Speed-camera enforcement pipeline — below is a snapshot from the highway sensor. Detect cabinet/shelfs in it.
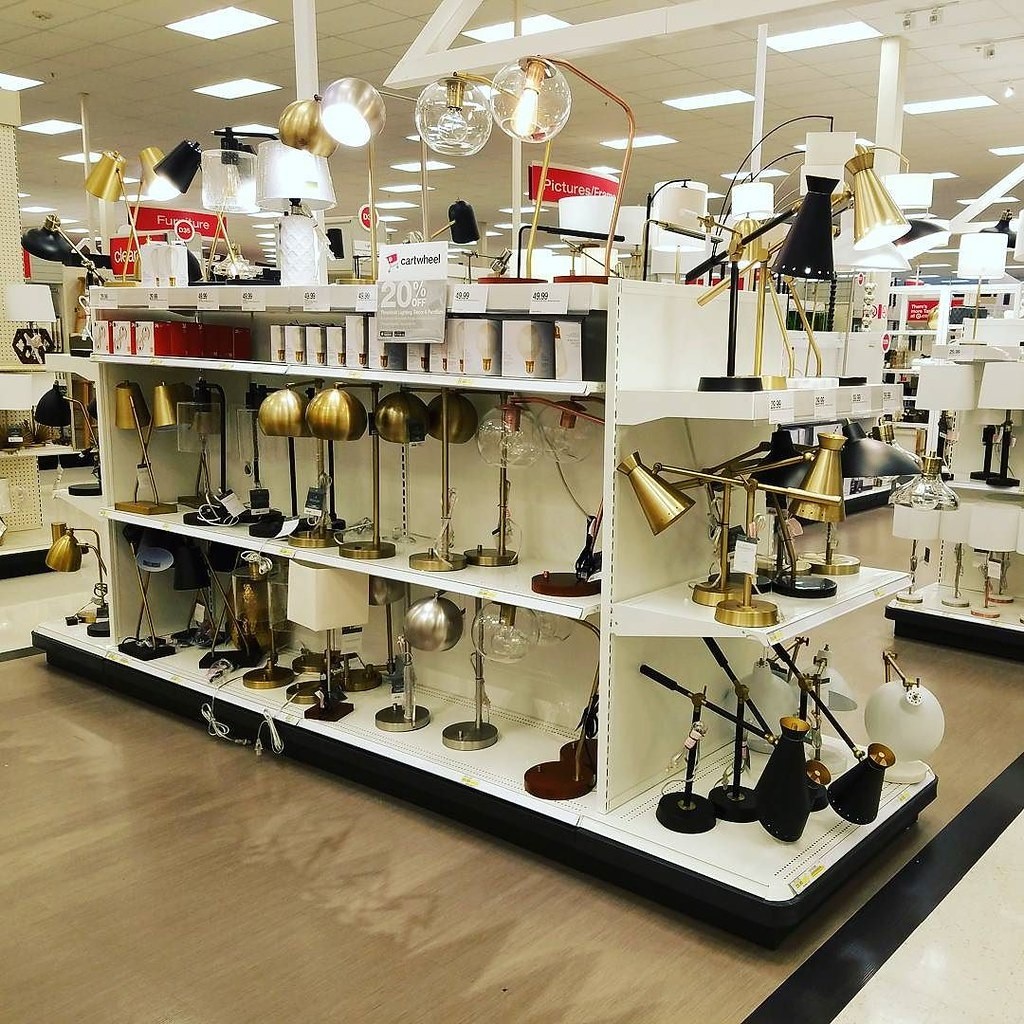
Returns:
[0,364,99,579]
[883,344,1024,665]
[884,284,1021,456]
[31,277,939,950]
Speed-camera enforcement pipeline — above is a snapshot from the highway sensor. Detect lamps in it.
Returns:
[0,54,1024,841]
